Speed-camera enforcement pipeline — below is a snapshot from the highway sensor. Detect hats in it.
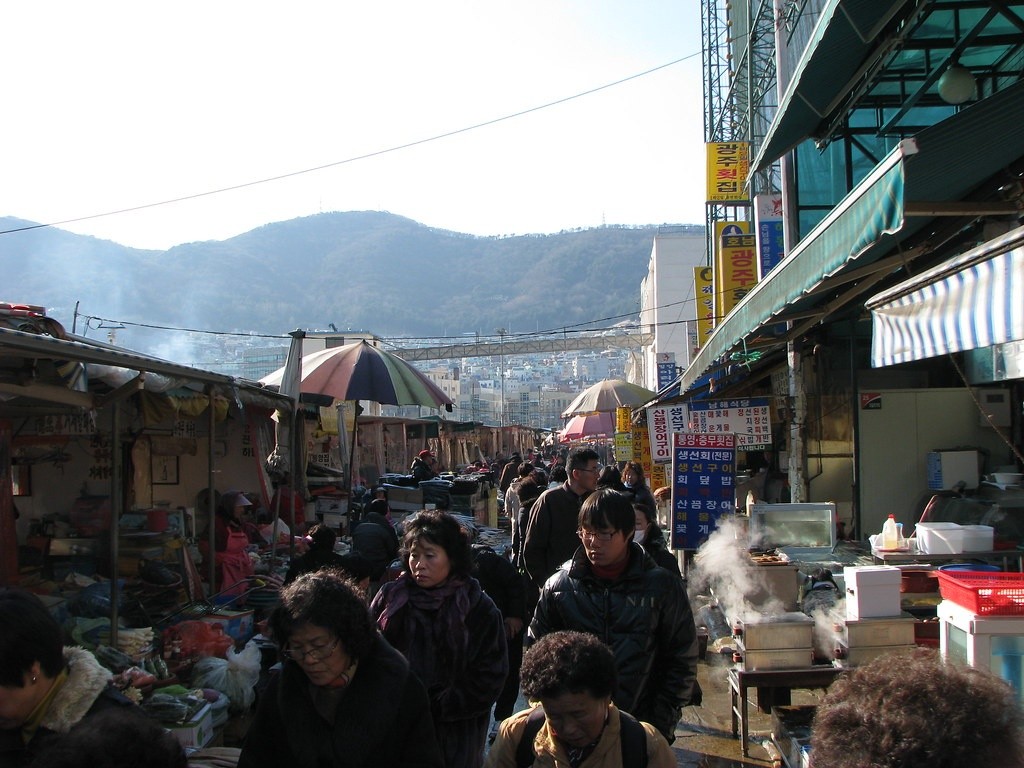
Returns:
[234,494,253,506]
[419,450,434,458]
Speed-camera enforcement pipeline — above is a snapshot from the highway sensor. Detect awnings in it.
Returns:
[864,224,1024,368]
[679,80,1024,397]
[742,0,912,193]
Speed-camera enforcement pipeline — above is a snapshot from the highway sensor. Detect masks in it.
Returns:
[632,525,650,544]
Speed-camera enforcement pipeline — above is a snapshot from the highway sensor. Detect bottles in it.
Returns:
[882,513,897,550]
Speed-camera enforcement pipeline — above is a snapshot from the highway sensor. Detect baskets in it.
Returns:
[933,570,1024,616]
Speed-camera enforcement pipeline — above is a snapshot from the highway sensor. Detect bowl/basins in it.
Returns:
[991,472,1023,484]
[899,570,939,593]
[939,563,1000,571]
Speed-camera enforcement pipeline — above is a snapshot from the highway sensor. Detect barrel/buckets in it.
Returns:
[145,508,168,532]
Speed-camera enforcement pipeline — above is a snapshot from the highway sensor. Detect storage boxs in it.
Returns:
[735,642,814,669]
[916,522,993,555]
[825,636,915,668]
[161,704,216,753]
[316,495,349,514]
[843,566,901,619]
[182,604,255,641]
[731,611,815,648]
[937,600,1024,711]
[828,611,918,646]
[750,503,837,555]
[324,513,348,528]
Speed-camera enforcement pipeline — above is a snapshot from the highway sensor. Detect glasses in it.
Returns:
[283,635,341,661]
[576,528,621,542]
[572,467,600,475]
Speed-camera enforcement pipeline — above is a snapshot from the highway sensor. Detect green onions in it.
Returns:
[98,627,154,647]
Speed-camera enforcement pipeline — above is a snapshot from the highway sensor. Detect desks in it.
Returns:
[727,666,859,752]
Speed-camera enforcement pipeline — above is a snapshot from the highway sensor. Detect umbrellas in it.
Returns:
[559,412,616,461]
[256,338,457,539]
[560,379,658,432]
[334,402,352,492]
[264,328,306,563]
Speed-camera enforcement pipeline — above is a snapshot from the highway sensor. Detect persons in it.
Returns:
[0,586,188,768]
[483,630,678,768]
[527,488,700,746]
[523,446,600,588]
[197,490,254,603]
[237,567,445,768]
[237,443,699,768]
[367,508,509,768]
